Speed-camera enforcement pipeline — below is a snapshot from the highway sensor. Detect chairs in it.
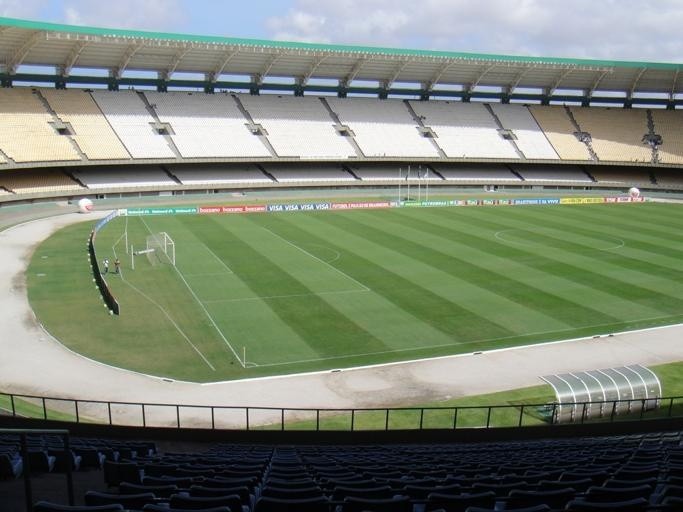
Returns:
[0,432,682,511]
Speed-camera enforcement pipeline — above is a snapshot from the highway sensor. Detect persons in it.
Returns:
[114,258,120,274]
[102,258,110,273]
